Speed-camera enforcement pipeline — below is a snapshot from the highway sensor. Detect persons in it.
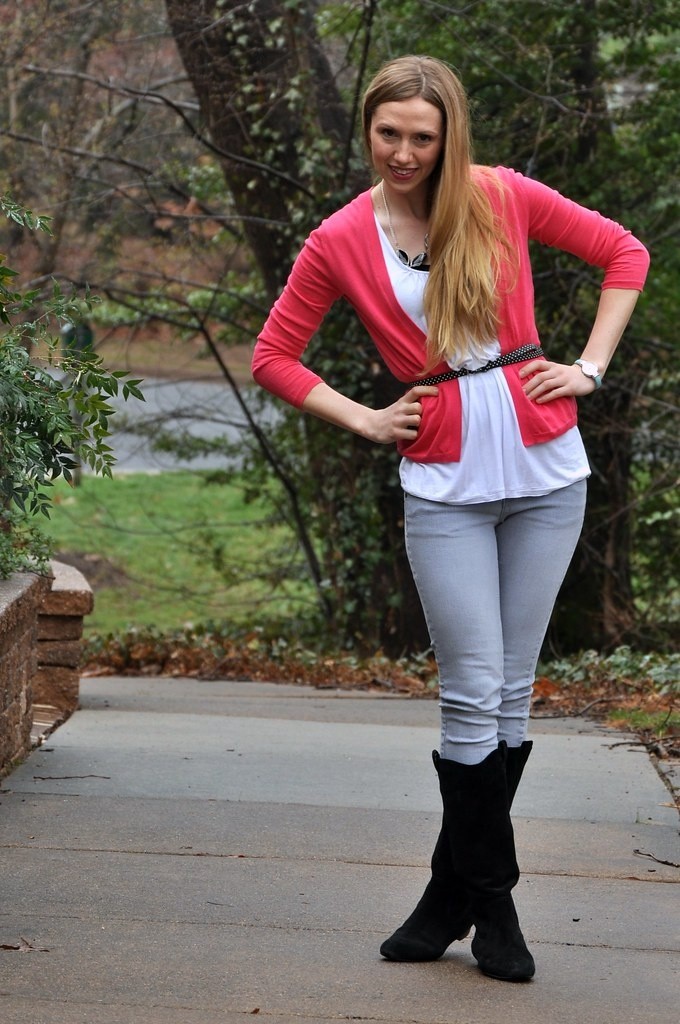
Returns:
[251,55,651,981]
[60,318,94,384]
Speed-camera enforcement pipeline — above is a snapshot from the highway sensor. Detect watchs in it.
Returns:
[574,360,602,390]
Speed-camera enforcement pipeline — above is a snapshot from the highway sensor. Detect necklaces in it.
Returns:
[380,179,400,249]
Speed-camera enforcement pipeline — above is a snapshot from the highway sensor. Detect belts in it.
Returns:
[403,344,544,390]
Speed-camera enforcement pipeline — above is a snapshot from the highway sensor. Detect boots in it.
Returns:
[432,741,536,985]
[379,738,535,962]
